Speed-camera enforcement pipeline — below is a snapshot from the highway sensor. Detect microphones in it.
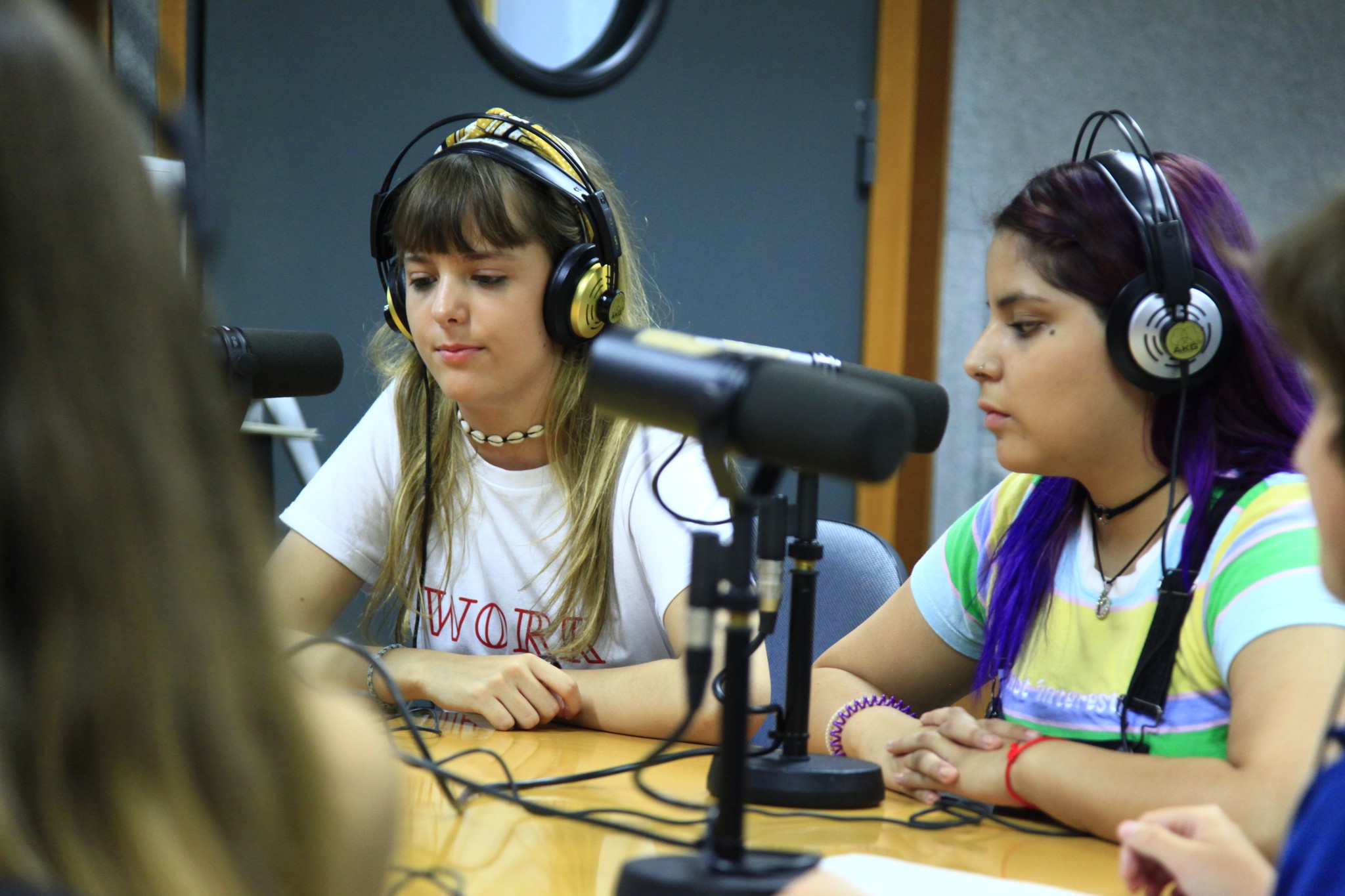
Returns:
[582,325,952,485]
[209,324,345,400]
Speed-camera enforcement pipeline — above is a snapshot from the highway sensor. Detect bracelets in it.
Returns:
[367,643,414,708]
[825,694,917,760]
[1004,737,1073,809]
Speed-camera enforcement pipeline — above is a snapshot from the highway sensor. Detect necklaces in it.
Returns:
[456,404,546,447]
[1088,480,1189,621]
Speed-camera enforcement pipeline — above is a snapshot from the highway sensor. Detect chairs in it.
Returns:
[734,518,898,717]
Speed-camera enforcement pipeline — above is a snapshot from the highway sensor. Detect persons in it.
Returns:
[1099,192,1345,896]
[262,104,774,748]
[1,0,413,896]
[796,144,1344,874]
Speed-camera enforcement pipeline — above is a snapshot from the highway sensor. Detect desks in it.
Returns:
[376,694,1170,896]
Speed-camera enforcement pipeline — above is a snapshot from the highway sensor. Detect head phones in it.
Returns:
[368,112,627,349]
[1072,110,1239,401]
